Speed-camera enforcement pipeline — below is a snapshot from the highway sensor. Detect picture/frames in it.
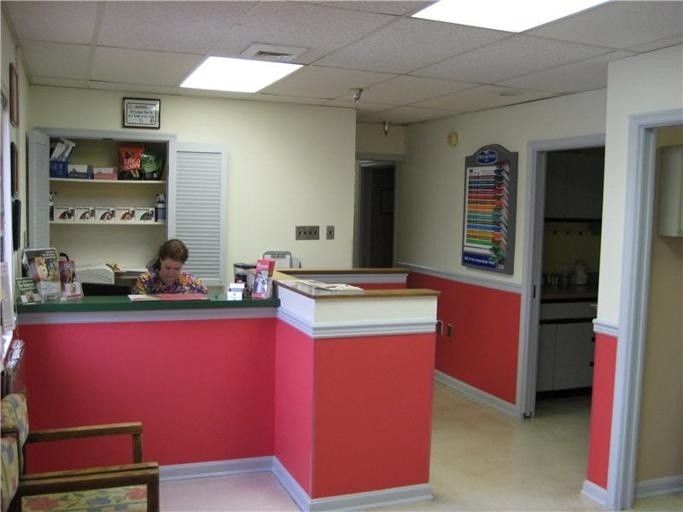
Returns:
[123,96,161,129]
[9,62,21,252]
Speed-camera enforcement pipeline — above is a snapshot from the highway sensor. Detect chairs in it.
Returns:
[0,392,159,512]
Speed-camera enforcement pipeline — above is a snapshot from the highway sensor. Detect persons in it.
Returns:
[129,238,208,295]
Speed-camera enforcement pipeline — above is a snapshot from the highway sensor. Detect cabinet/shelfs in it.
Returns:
[535,297,598,401]
[27,126,227,288]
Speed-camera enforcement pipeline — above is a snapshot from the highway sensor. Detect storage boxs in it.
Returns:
[68,165,118,181]
